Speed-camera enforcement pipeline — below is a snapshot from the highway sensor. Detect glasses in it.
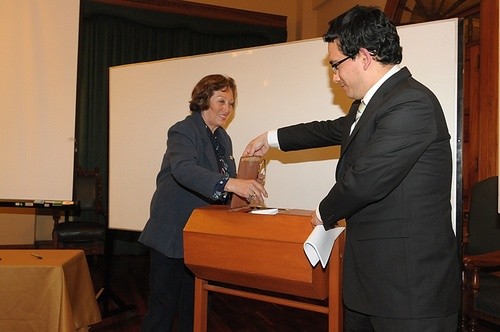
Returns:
[330,52,359,73]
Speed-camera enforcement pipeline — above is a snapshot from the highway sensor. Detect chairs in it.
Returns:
[52,164,107,318]
[462,173,500,331]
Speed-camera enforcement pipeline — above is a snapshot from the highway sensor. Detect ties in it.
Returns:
[349,101,367,135]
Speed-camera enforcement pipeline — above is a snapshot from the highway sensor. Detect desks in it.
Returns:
[0,249,102,332]
[0,199,80,249]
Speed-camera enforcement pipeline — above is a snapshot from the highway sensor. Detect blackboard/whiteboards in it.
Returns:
[108,18,464,238]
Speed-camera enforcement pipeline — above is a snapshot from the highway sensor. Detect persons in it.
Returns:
[138,74,268,332]
[242,6,462,332]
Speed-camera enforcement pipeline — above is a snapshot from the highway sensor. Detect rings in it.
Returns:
[248,192,256,201]
